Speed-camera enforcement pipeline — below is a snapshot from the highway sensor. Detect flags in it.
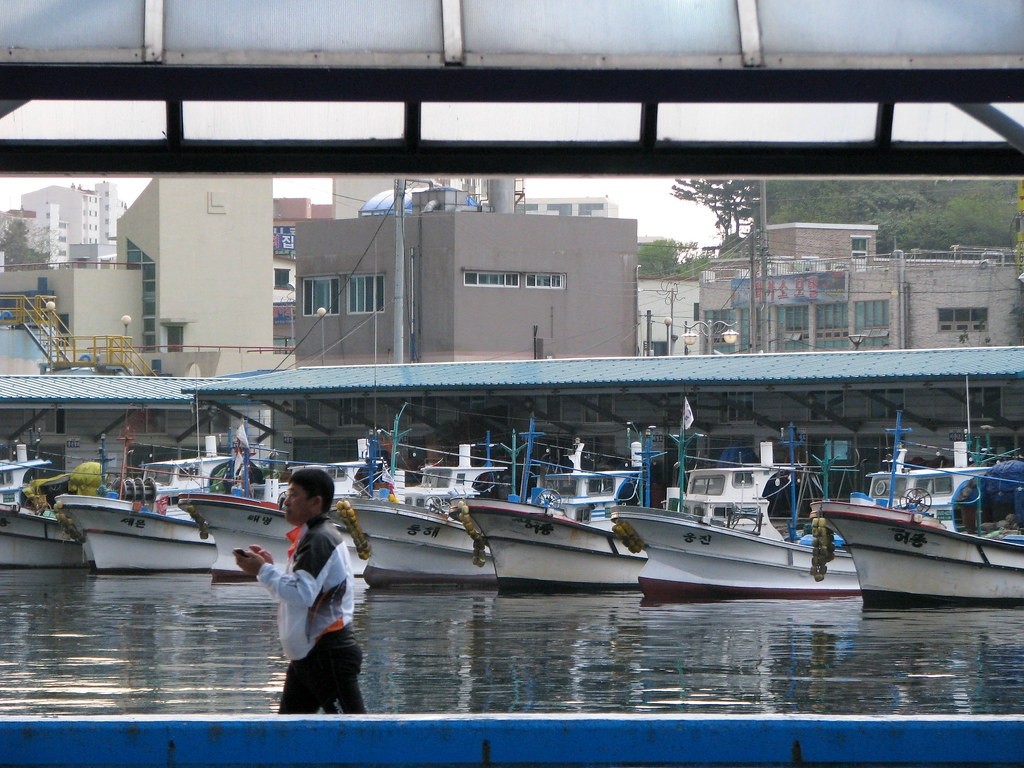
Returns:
[685,396,694,430]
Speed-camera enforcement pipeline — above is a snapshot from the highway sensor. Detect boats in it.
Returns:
[2,388,1024,615]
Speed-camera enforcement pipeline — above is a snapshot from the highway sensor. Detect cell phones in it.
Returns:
[233,547,249,558]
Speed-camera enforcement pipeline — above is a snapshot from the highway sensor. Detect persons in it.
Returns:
[232,469,366,715]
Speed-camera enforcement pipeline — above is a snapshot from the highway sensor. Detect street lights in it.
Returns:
[664,317,673,356]
[46,301,56,375]
[681,320,739,355]
[848,334,867,350]
[120,314,132,364]
[317,306,327,367]
